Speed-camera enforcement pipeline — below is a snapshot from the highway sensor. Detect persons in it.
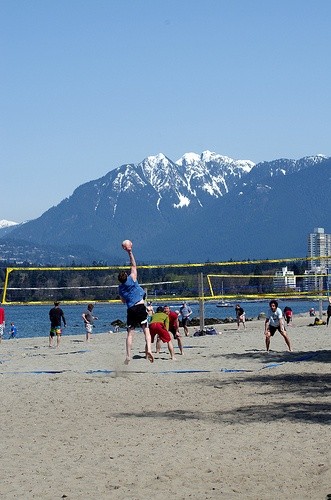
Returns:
[48,302,66,347]
[117,242,153,365]
[264,300,292,352]
[283,306,293,325]
[235,304,246,331]
[0,302,5,344]
[146,301,155,323]
[82,304,98,341]
[178,302,192,336]
[8,322,18,340]
[155,305,184,356]
[145,307,177,360]
[312,317,326,326]
[326,301,331,327]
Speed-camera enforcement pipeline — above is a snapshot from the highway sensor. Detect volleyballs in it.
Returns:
[123,240,133,250]
